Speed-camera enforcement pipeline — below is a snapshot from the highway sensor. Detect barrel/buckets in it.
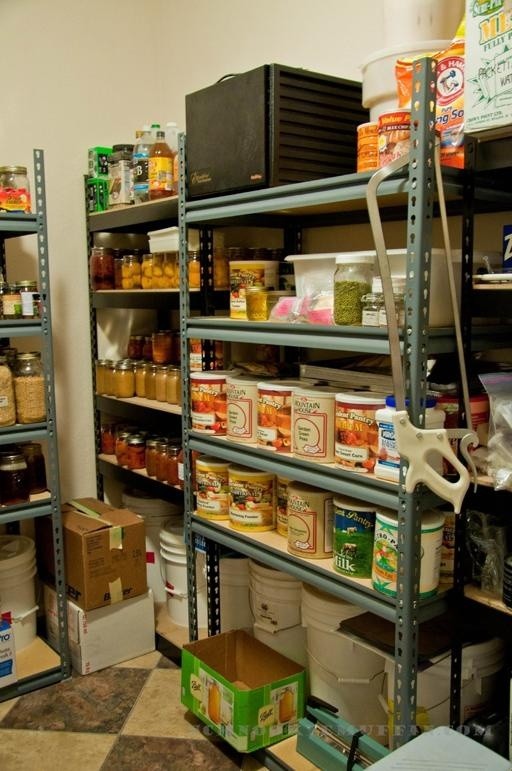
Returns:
[205,553,253,637]
[119,491,186,606]
[299,580,395,754]
[159,516,210,631]
[384,635,510,753]
[245,557,308,720]
[1,533,38,655]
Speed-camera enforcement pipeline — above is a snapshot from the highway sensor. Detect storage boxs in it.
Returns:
[44,584,155,674]
[181,628,305,753]
[35,496,147,610]
[286,249,462,327]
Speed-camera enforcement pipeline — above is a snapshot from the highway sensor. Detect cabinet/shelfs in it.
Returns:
[84,132,193,668]
[0,149,71,704]
[451,124,512,755]
[179,56,453,771]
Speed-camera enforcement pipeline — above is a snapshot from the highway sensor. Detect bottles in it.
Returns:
[0,165,31,213]
[469,393,490,474]
[433,382,459,472]
[279,686,295,723]
[332,493,379,578]
[287,480,334,559]
[334,389,393,474]
[266,290,296,319]
[0,353,15,427]
[88,244,229,289]
[0,453,30,505]
[195,454,231,520]
[360,292,404,326]
[1,279,39,318]
[21,442,47,493]
[257,378,312,453]
[188,367,241,435]
[229,463,276,533]
[333,253,375,325]
[106,145,134,205]
[356,121,379,171]
[290,385,345,461]
[131,121,178,203]
[378,108,418,169]
[190,340,224,372]
[225,375,260,442]
[228,246,287,261]
[207,681,221,726]
[244,285,275,320]
[229,259,280,319]
[94,327,181,407]
[372,275,405,293]
[373,394,448,484]
[275,474,293,537]
[371,508,446,596]
[99,419,185,486]
[13,350,46,423]
